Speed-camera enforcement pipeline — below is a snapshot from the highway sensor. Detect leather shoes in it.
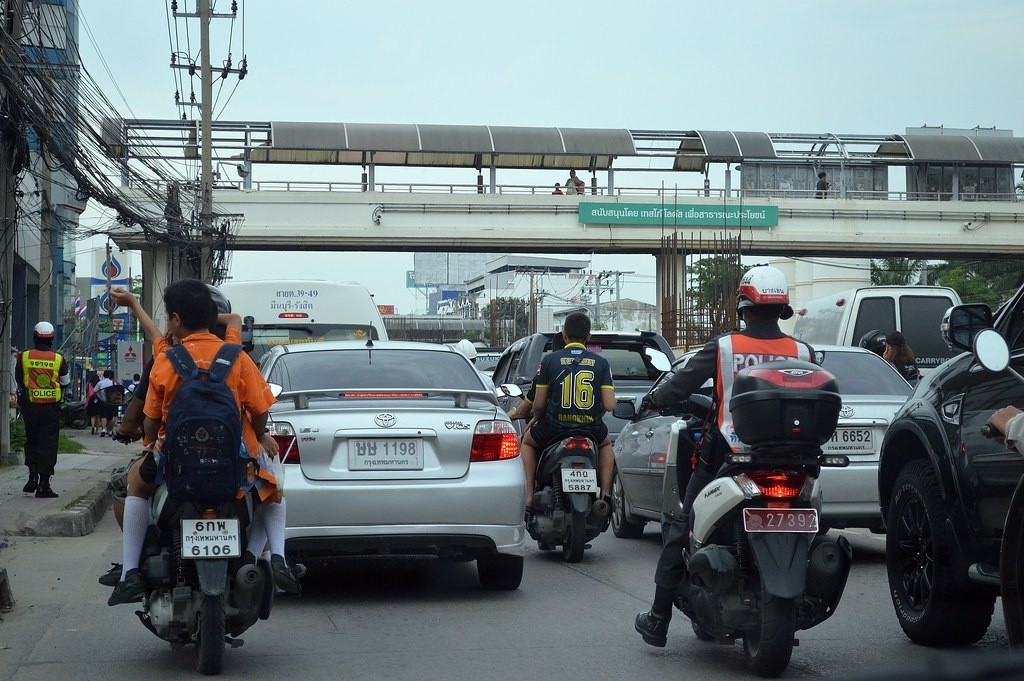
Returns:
[635,610,670,647]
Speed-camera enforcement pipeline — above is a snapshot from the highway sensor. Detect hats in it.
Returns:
[886,331,906,346]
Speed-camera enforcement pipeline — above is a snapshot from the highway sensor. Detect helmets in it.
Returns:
[859,330,888,355]
[738,266,789,304]
[204,284,230,313]
[33,321,54,337]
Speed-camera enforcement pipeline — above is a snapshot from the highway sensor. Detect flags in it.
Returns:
[74,294,88,319]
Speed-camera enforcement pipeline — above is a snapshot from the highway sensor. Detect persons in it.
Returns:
[552,183,564,195]
[882,331,923,381]
[521,313,617,509]
[984,405,1024,460]
[565,170,582,196]
[506,332,567,449]
[634,265,816,648]
[83,277,302,606]
[14,321,70,500]
[454,339,498,404]
[814,172,830,200]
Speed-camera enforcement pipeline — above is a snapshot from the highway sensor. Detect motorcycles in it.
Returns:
[57,400,87,428]
[95,380,283,675]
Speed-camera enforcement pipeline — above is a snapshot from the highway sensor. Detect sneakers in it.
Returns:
[270,559,302,593]
[23,475,38,493]
[98,563,122,586]
[291,564,306,577]
[35,483,59,498]
[108,573,148,606]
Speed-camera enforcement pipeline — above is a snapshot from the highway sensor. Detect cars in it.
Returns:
[875,282,1024,651]
[444,341,510,379]
[491,328,678,453]
[255,341,531,592]
[610,345,915,541]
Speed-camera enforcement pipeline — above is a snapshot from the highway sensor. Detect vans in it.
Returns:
[789,285,965,390]
[215,279,387,370]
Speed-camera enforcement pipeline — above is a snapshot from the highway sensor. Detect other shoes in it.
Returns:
[91,427,114,437]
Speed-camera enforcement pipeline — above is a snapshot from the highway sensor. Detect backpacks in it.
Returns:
[155,344,259,499]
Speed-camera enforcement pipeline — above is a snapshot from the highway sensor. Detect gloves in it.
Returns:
[643,394,656,408]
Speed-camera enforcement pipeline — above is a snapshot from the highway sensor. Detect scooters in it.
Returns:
[637,345,854,675]
[973,327,1024,659]
[499,385,610,564]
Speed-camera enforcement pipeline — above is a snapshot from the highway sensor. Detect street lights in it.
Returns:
[199,159,251,280]
